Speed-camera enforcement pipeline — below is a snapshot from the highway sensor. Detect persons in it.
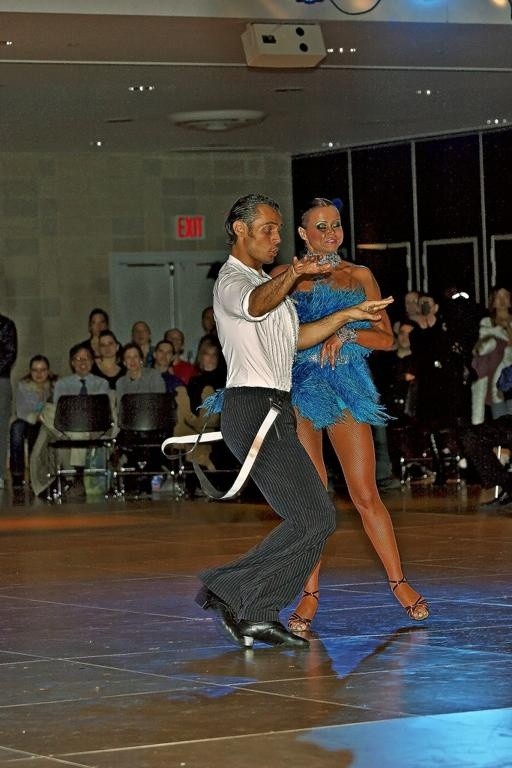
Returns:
[194,193,396,648]
[1,288,512,510]
[269,195,430,634]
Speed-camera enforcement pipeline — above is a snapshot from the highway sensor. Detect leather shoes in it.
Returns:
[13,475,23,486]
[195,583,242,645]
[237,619,310,649]
[480,490,511,508]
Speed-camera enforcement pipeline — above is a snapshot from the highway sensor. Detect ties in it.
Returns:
[80,379,87,395]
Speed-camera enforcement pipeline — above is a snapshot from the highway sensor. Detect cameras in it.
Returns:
[422,303,431,317]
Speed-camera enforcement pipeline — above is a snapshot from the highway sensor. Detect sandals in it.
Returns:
[388,576,428,621]
[287,589,320,632]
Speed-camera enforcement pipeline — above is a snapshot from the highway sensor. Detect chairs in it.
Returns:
[112,393,183,502]
[47,393,115,507]
